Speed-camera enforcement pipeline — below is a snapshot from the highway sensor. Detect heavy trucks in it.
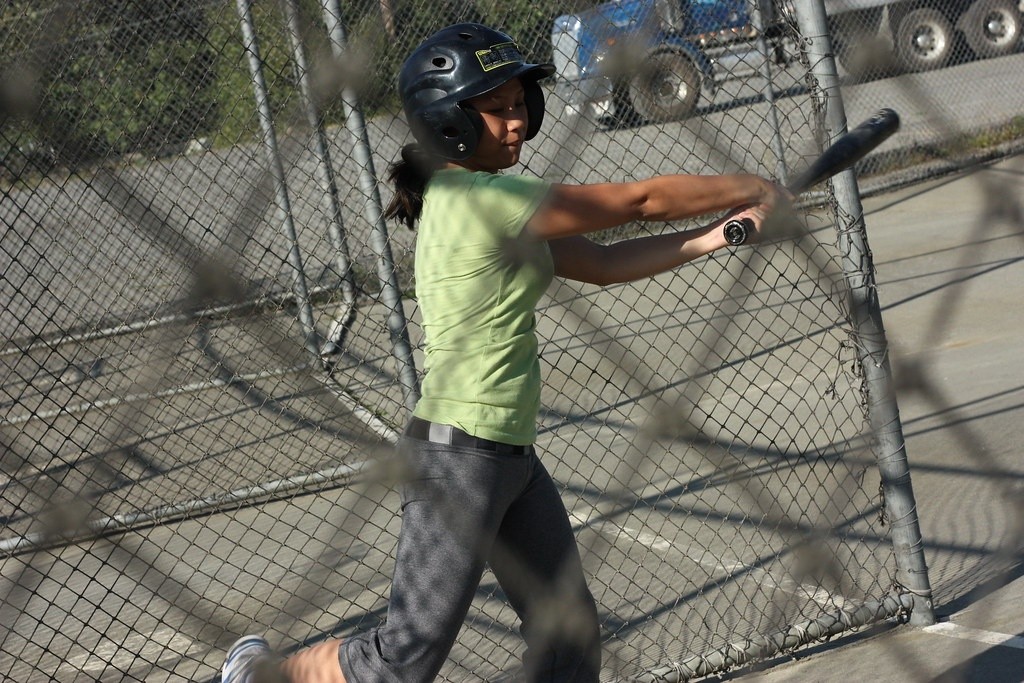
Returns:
[552,0,1024,128]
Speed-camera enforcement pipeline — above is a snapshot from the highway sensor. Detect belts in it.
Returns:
[405,417,534,455]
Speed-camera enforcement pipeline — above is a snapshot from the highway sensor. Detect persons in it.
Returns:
[221,23,796,683]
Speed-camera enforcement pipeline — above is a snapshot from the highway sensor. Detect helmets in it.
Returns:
[400,21,558,162]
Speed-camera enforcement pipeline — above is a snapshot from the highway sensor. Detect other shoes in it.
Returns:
[222,635,270,683]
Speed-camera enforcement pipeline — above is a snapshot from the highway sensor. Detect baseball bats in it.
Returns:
[722,106,902,249]
